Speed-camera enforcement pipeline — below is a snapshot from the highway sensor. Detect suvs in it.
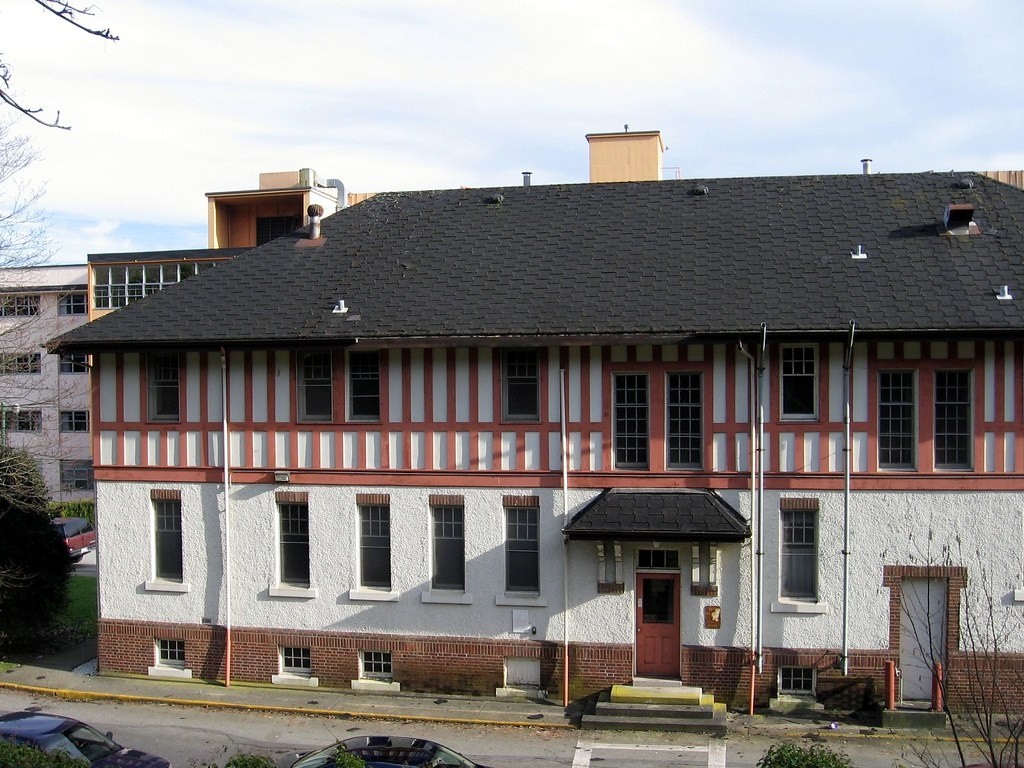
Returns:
[51,518,97,563]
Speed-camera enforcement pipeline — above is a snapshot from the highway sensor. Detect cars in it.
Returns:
[274,734,494,768]
[0,711,172,768]
